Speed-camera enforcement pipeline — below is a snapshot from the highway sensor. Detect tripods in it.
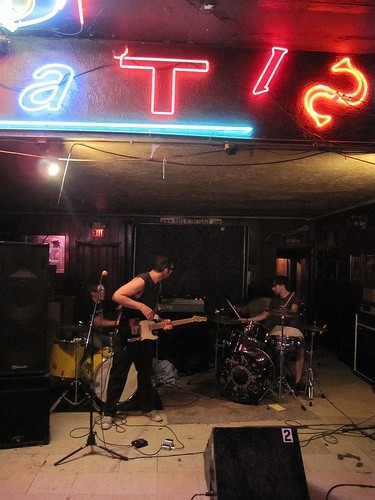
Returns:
[53,278,127,466]
[257,317,306,412]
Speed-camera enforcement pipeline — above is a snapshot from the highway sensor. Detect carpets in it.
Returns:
[157,369,320,423]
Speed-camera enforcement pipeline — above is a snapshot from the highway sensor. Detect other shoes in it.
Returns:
[293,379,304,393]
[151,410,163,422]
[101,415,113,429]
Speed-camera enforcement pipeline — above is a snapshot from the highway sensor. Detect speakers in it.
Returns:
[0,240,50,451]
[202,426,309,500]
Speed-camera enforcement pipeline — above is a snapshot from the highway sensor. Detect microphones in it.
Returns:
[100,270,107,280]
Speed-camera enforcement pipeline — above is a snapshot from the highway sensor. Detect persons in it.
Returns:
[100,253,174,431]
[74,283,116,349]
[239,276,311,393]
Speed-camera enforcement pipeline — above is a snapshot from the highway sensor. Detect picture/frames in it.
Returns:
[314,254,375,285]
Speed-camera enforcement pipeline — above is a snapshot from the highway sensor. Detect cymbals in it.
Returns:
[56,325,89,331]
[209,316,244,325]
[303,325,327,332]
[271,310,299,317]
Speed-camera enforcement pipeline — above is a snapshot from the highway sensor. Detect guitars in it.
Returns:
[128,315,207,343]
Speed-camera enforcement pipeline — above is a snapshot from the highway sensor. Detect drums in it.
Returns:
[267,335,300,352]
[218,346,275,404]
[81,346,139,405]
[238,320,268,347]
[48,338,90,381]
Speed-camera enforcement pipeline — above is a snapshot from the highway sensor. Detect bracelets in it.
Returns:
[246,318,248,322]
